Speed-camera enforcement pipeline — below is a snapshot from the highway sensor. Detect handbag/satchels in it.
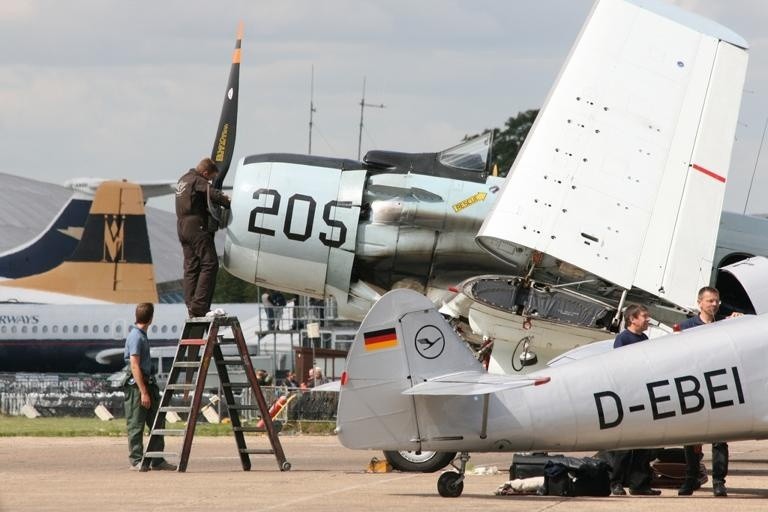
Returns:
[219,208,229,229]
[543,460,613,496]
[508,451,565,480]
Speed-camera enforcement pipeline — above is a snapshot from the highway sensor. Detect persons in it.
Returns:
[176,159,232,319]
[254,366,327,388]
[123,303,177,470]
[609,304,663,496]
[677,286,744,497]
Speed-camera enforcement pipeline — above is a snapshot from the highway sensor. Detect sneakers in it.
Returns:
[712,476,728,496]
[678,470,708,494]
[150,460,177,471]
[629,487,661,495]
[127,460,152,470]
[610,481,626,495]
[190,308,226,318]
